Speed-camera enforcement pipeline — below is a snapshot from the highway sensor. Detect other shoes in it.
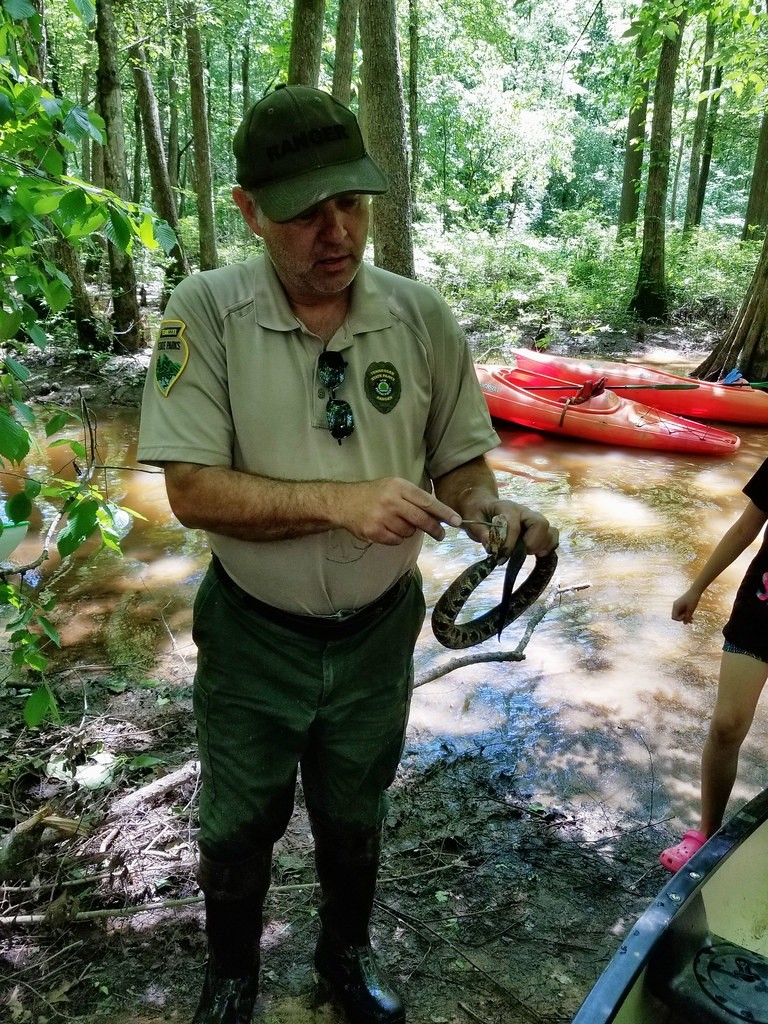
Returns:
[192,965,258,1024]
[314,930,406,1022]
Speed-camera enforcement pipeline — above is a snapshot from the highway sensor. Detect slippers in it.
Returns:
[659,828,707,873]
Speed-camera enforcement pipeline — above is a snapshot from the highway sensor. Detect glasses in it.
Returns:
[318,353,356,448]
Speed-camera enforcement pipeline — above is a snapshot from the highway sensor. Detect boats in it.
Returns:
[511,347,768,428]
[474,362,742,457]
[571,787,768,1023]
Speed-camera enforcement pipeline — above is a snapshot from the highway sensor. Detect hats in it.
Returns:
[233,82,390,225]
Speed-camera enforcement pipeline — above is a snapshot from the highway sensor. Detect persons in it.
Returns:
[659,454,768,873]
[136,83,559,1024]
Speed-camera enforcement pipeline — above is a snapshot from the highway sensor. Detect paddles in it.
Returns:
[521,382,701,391]
[720,381,768,390]
